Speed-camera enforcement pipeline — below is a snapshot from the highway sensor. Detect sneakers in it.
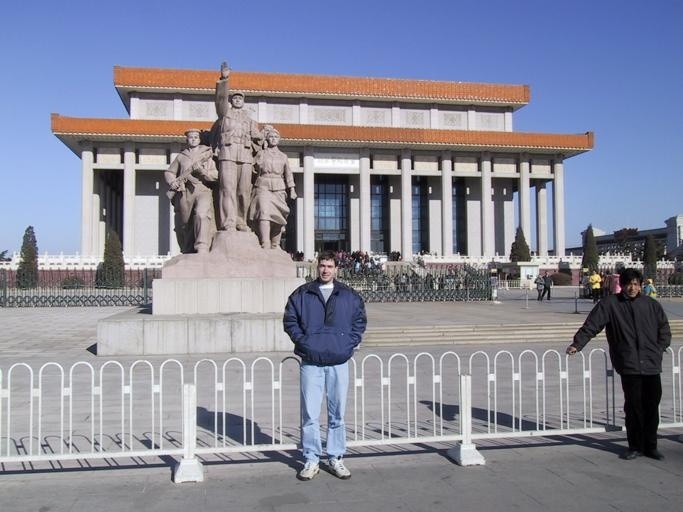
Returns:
[327,457,351,479]
[296,461,320,481]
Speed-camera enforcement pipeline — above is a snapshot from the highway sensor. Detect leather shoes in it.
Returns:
[644,449,664,460]
[618,449,641,460]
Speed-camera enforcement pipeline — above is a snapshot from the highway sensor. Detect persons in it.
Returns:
[564,268,672,459]
[252,123,298,250]
[541,271,553,299]
[582,267,657,305]
[213,61,264,232]
[164,129,218,253]
[282,250,367,481]
[533,273,545,300]
[288,248,512,292]
